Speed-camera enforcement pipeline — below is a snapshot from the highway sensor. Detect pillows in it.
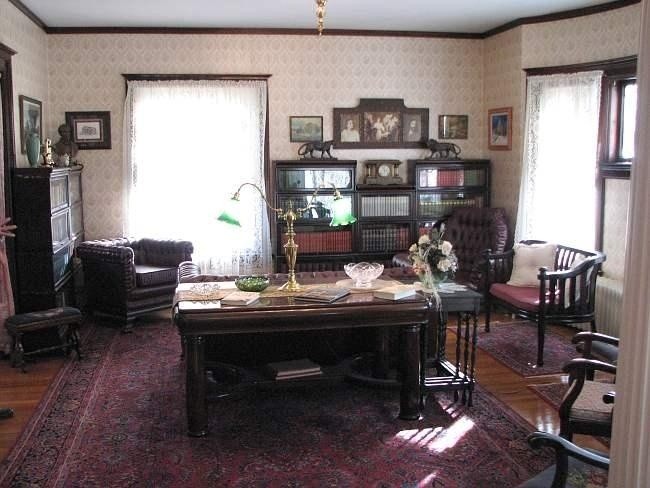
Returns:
[505,241,559,291]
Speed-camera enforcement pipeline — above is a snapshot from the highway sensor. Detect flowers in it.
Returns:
[407,217,460,274]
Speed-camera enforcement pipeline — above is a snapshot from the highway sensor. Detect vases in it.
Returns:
[413,264,449,289]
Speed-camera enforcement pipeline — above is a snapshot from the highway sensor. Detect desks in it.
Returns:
[395,271,485,411]
[173,265,431,436]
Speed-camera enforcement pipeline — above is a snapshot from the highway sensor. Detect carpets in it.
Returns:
[0,322,613,487]
[524,377,616,452]
[443,317,601,379]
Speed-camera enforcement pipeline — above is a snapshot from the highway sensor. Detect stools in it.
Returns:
[4,306,84,374]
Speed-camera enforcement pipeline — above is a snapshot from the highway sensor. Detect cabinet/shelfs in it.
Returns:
[354,183,416,254]
[272,161,358,255]
[10,163,85,314]
[409,158,491,251]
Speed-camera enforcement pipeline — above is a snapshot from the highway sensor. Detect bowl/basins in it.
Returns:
[235,276,270,292]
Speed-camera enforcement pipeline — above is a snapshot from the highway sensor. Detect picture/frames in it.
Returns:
[64,110,112,151]
[437,114,469,140]
[17,94,43,155]
[289,115,323,143]
[487,106,512,151]
[331,97,430,149]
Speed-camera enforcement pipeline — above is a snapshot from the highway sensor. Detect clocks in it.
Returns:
[363,160,403,186]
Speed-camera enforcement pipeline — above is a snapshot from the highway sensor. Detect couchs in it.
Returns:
[392,205,509,319]
[74,235,195,334]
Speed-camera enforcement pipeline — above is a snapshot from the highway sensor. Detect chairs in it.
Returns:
[474,237,608,368]
[522,431,609,488]
[556,329,620,443]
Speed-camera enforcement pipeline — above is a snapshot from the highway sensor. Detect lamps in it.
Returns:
[216,181,357,293]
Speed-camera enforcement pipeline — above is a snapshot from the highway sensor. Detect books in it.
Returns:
[359,196,409,217]
[294,284,351,303]
[222,290,259,308]
[269,360,323,380]
[373,284,415,300]
[421,168,485,188]
[359,227,411,251]
[280,227,351,254]
[420,195,483,218]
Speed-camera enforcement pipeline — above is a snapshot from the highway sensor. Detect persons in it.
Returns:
[341,115,361,143]
[0,214,17,420]
[371,117,386,142]
[404,118,422,142]
[55,124,78,156]
